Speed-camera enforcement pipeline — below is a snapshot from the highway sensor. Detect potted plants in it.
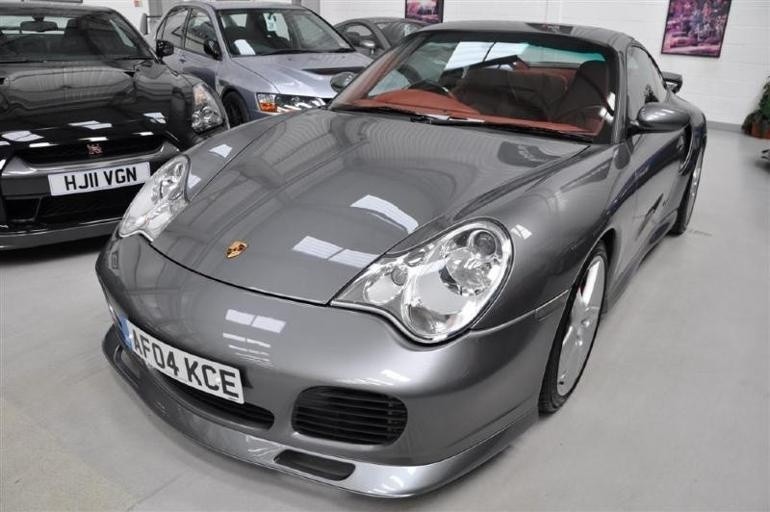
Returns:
[741,76,770,139]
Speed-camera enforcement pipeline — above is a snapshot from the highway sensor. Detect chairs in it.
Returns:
[199,21,246,41]
[439,60,611,132]
[347,31,360,45]
[1,14,100,55]
